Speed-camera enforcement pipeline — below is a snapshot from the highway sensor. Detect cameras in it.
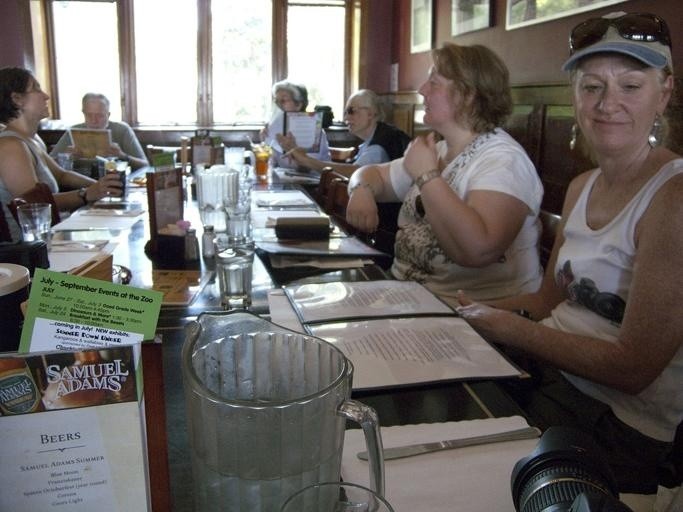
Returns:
[510,424,634,512]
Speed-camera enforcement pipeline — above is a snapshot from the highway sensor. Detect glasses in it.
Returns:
[571,11,672,49]
[343,106,369,115]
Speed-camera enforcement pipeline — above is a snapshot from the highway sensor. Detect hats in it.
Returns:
[561,11,675,75]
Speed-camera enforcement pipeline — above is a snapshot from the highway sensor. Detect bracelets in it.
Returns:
[349,183,374,196]
[282,146,297,163]
[125,156,132,163]
[415,169,441,189]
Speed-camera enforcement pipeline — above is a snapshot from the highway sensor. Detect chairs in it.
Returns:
[4,178,61,238]
[314,141,379,249]
[536,207,564,277]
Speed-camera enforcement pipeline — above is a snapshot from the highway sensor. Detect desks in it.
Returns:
[0,160,606,510]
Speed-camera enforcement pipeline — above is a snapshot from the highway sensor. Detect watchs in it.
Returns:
[78,187,88,205]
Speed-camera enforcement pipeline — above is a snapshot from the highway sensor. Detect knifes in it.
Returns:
[355,426,542,463]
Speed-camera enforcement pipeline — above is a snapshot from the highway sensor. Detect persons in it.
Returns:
[0,67,124,240]
[258,79,332,174]
[49,92,150,169]
[453,11,683,496]
[275,88,413,271]
[344,41,545,311]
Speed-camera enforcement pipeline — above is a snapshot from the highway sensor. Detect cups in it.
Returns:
[280,482,394,511]
[16,202,53,252]
[106,168,126,202]
[193,165,255,311]
[254,152,268,177]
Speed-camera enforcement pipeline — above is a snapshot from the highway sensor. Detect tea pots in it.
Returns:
[180,308,385,511]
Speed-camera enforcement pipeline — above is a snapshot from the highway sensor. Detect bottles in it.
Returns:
[187,231,201,265]
[0,357,44,415]
[0,261,30,353]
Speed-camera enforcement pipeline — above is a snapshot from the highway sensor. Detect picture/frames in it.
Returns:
[408,0,434,55]
[502,0,628,32]
[448,0,495,38]
